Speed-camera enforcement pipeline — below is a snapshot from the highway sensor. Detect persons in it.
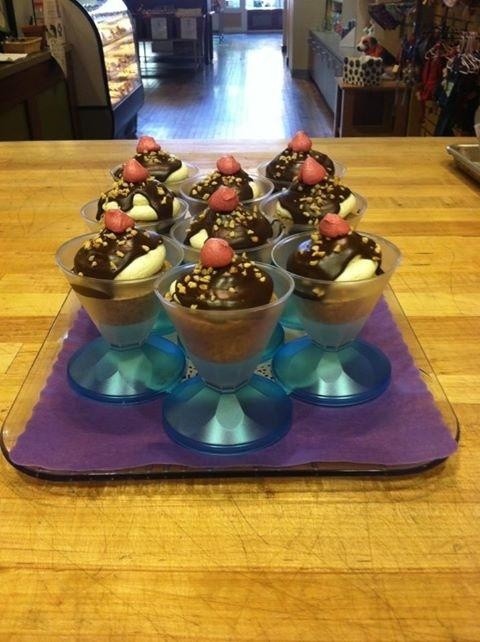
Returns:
[363,3,402,58]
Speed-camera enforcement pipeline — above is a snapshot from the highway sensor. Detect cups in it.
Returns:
[152,261,294,451]
[109,158,198,188]
[256,158,347,186]
[80,197,190,336]
[180,172,275,211]
[55,230,187,411]
[170,215,286,366]
[271,231,401,410]
[260,187,368,333]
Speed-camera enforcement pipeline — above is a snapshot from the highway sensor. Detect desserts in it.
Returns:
[188,157,263,203]
[115,136,188,184]
[96,160,181,224]
[274,155,360,226]
[67,211,167,300]
[287,213,384,300]
[165,238,273,321]
[180,188,276,251]
[266,132,335,183]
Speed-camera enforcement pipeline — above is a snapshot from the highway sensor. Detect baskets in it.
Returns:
[0,36,43,54]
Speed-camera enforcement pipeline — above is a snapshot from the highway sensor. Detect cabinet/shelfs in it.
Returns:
[303,25,345,115]
[133,12,206,80]
[244,0,284,32]
[222,0,244,31]
[0,45,74,142]
